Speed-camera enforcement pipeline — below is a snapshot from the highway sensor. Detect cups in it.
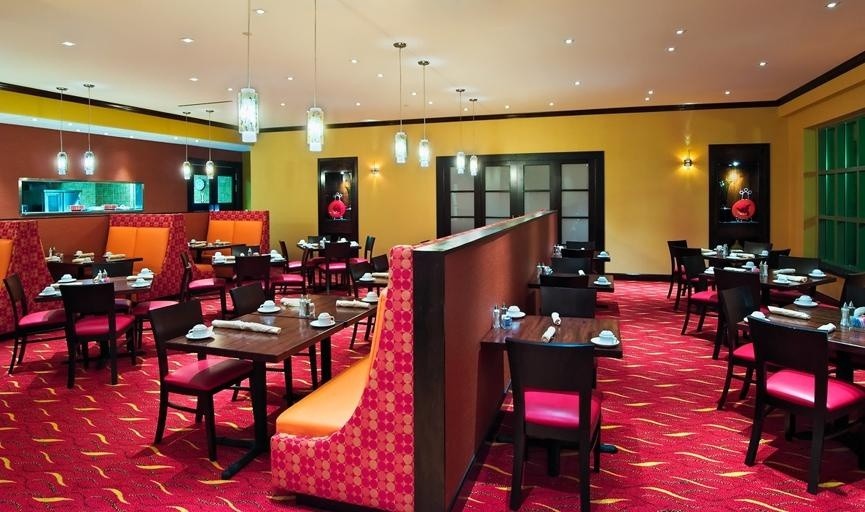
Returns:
[318,313,334,324]
[597,276,608,283]
[189,323,209,337]
[62,273,72,280]
[260,300,276,310]
[367,291,377,300]
[508,305,520,315]
[42,287,55,293]
[812,269,823,275]
[797,295,812,303]
[746,261,754,267]
[362,272,372,279]
[778,276,788,281]
[600,251,608,256]
[762,250,768,254]
[599,330,617,343]
[310,320,335,328]
[591,337,620,346]
[758,253,769,256]
[793,301,818,307]
[141,268,151,274]
[136,278,147,284]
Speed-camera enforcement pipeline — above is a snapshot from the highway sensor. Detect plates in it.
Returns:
[58,279,77,282]
[593,280,611,285]
[362,297,379,302]
[359,278,375,281]
[257,306,281,313]
[772,280,791,284]
[742,265,757,268]
[597,255,610,258]
[507,312,525,318]
[137,273,154,275]
[808,273,827,278]
[186,333,212,340]
[131,283,151,288]
[38,293,57,296]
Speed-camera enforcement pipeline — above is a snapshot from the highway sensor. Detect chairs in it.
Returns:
[743,314,865,495]
[673,244,724,336]
[232,255,276,301]
[503,336,605,512]
[268,246,314,298]
[712,266,761,359]
[837,270,865,324]
[710,284,801,443]
[348,233,374,264]
[53,282,139,390]
[228,280,319,406]
[665,238,705,313]
[178,249,231,316]
[538,273,590,287]
[767,246,791,270]
[561,247,595,259]
[316,239,353,294]
[365,253,391,278]
[777,251,823,301]
[1,271,69,374]
[538,286,597,318]
[550,255,590,273]
[347,260,381,348]
[145,297,258,463]
[564,239,598,253]
[743,238,775,259]
[305,233,334,251]
[279,239,312,295]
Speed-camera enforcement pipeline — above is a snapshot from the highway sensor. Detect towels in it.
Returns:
[772,267,797,275]
[577,268,586,277]
[776,273,809,285]
[71,257,93,263]
[729,248,744,254]
[723,265,747,273]
[48,282,84,287]
[334,298,371,309]
[550,304,563,326]
[729,252,755,259]
[211,317,283,335]
[262,252,282,257]
[73,252,96,257]
[45,255,61,263]
[767,305,811,320]
[370,271,390,278]
[214,240,232,246]
[108,252,128,260]
[701,250,718,256]
[280,296,305,306]
[816,321,837,334]
[540,324,557,343]
[126,274,154,279]
[190,237,207,248]
[211,254,238,260]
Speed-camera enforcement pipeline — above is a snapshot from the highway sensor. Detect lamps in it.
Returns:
[204,109,217,182]
[469,98,479,177]
[393,42,408,165]
[176,110,193,182]
[52,86,71,176]
[456,88,466,175]
[680,136,706,173]
[80,82,98,176]
[417,60,431,169]
[304,1,326,153]
[235,0,260,145]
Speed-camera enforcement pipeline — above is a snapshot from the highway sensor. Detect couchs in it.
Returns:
[196,209,274,284]
[91,210,216,303]
[266,208,560,510]
[0,218,68,338]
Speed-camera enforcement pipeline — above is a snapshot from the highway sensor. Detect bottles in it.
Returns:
[759,261,764,277]
[501,303,507,315]
[841,302,850,327]
[542,262,544,268]
[848,301,855,321]
[98,270,103,279]
[299,294,305,314]
[536,262,541,273]
[103,269,107,278]
[493,305,500,328]
[763,261,768,277]
[49,248,52,257]
[53,247,56,255]
[305,294,310,316]
